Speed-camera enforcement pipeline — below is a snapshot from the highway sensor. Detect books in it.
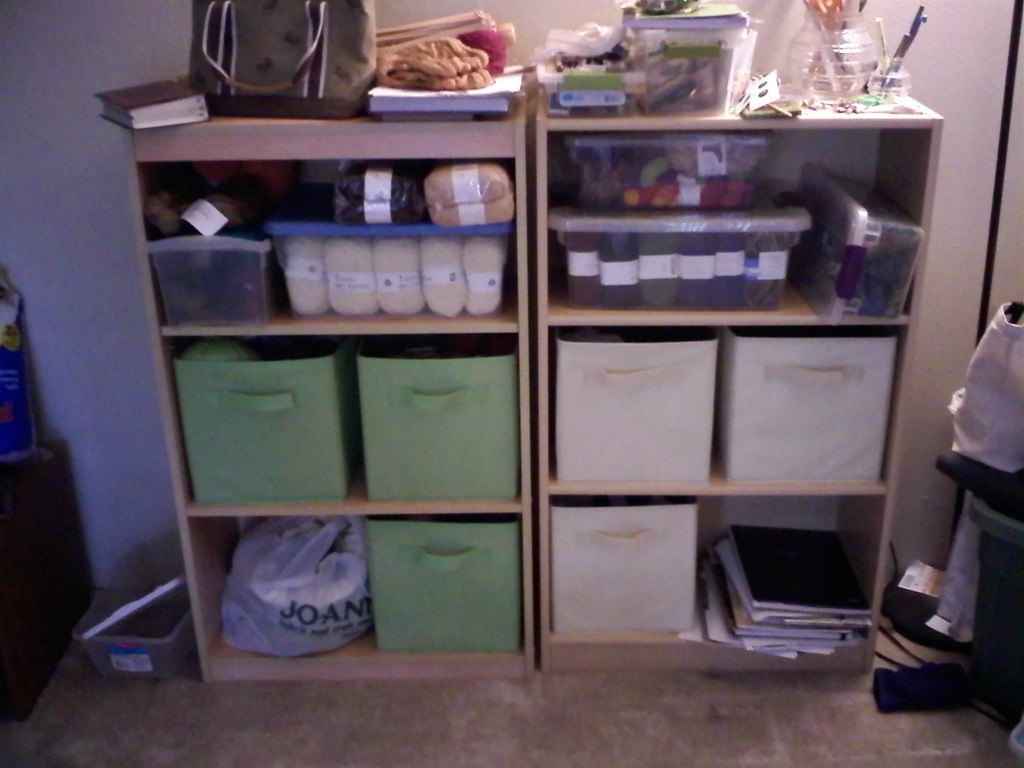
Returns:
[700,526,874,656]
[91,77,210,133]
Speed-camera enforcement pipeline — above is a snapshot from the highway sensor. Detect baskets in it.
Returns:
[71,571,196,677]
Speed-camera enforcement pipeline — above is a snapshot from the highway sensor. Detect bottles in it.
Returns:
[867,59,911,100]
[785,12,880,106]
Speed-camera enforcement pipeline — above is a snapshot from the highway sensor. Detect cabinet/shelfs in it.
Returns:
[0,438,94,723]
[132,84,535,681]
[533,90,944,673]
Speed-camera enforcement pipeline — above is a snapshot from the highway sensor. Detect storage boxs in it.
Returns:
[537,60,645,116]
[143,164,924,653]
[631,25,759,113]
[971,494,1024,718]
[72,544,196,678]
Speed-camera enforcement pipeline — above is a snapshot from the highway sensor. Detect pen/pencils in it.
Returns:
[874,5,928,97]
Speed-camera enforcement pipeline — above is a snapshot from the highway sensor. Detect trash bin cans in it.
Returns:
[966,494,1024,724]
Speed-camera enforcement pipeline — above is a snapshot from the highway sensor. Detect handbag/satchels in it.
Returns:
[221,513,372,656]
[189,0,376,119]
[949,297,1024,473]
